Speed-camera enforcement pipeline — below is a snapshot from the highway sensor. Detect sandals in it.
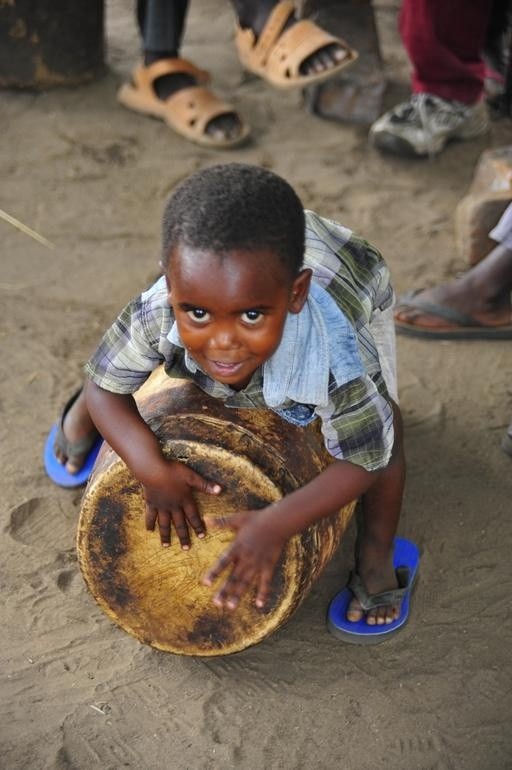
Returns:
[233,1,359,92]
[114,57,251,149]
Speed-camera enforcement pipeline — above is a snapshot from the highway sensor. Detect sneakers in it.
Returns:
[369,94,490,157]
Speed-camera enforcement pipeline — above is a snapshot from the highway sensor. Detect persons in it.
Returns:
[366,1,511,162]
[110,0,360,147]
[42,160,424,647]
[391,200,512,341]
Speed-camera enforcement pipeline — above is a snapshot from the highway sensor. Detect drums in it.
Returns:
[76,361,358,657]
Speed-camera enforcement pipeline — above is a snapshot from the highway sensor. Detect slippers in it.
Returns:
[393,288,512,338]
[42,384,103,489]
[326,538,419,646]
